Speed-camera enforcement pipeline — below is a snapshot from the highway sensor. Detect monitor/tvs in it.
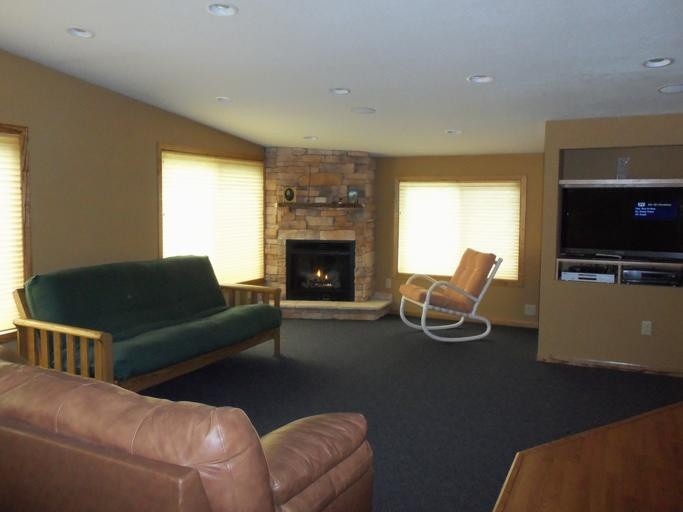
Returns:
[558,183,683,260]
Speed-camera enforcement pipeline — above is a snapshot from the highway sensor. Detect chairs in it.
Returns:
[399,248,502,343]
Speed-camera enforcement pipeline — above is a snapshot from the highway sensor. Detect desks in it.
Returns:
[490,397,683,512]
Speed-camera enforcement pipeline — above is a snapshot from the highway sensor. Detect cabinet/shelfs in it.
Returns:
[554,257,683,287]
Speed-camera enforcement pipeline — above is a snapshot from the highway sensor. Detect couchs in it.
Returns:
[0,358,372,511]
[12,255,281,393]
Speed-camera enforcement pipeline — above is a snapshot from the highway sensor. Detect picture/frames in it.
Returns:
[347,188,359,202]
[282,185,297,203]
